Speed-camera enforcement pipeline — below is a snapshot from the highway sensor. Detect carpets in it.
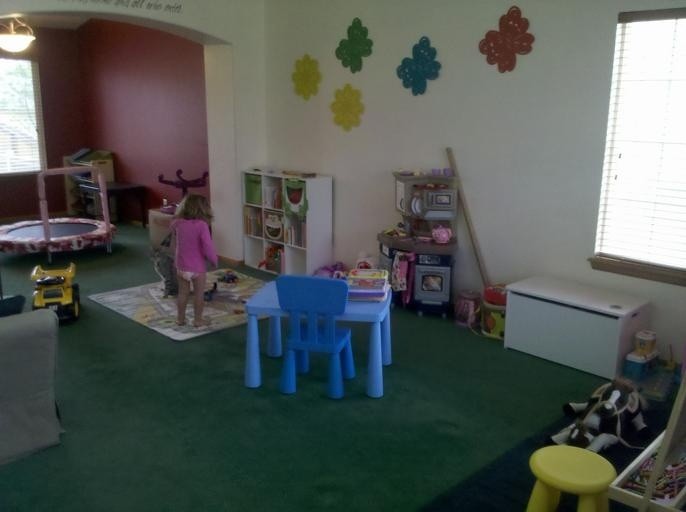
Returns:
[422,404,671,512]
[88,267,273,342]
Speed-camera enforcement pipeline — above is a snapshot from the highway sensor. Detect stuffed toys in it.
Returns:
[548,373,652,455]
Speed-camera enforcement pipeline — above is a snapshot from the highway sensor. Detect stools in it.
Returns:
[158,169,208,213]
[526,445,617,512]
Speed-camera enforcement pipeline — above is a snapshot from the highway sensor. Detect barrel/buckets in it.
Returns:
[477,299,506,340]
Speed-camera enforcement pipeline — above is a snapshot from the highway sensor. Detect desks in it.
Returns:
[80,182,146,229]
[149,209,173,246]
[506,278,650,379]
[245,279,392,398]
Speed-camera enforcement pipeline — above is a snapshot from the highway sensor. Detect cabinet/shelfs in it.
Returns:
[241,170,332,275]
[62,156,116,221]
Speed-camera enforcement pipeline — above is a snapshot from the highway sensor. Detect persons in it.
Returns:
[166,193,222,331]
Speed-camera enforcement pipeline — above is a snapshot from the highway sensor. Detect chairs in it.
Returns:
[275,275,355,399]
[0,293,62,467]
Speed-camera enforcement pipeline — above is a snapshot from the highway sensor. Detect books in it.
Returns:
[342,267,389,302]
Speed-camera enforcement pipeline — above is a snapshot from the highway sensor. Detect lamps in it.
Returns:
[0,17,36,52]
